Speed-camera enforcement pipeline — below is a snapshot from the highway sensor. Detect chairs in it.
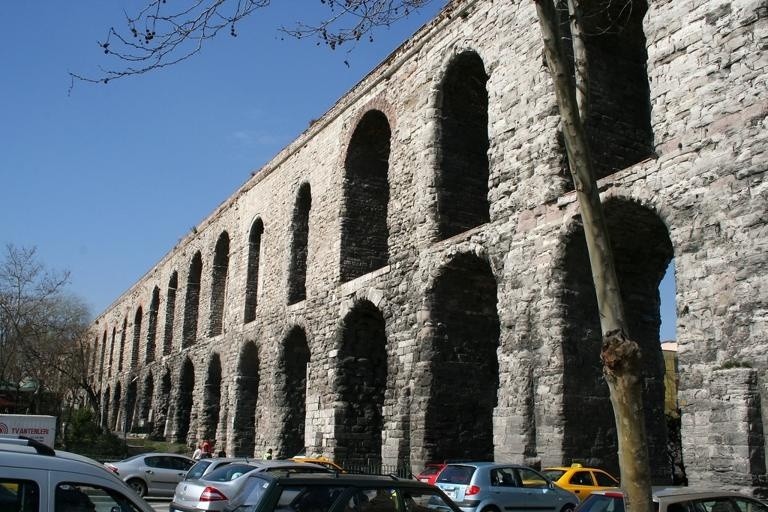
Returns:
[502,473,516,487]
[369,496,395,512]
[712,502,733,512]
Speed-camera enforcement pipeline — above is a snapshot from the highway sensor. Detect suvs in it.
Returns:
[0,433,157,512]
[222,472,463,512]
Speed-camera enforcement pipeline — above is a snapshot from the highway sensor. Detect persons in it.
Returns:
[190,442,194,457]
[264,448,274,460]
[201,435,211,453]
[193,445,201,462]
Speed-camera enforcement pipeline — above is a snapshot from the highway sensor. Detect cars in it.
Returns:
[571,487,768,512]
[435,462,582,512]
[101,453,198,501]
[521,463,622,503]
[416,463,469,486]
[184,458,259,484]
[167,460,338,511]
[287,454,347,473]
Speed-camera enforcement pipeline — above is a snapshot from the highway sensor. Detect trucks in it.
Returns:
[0,414,56,449]
